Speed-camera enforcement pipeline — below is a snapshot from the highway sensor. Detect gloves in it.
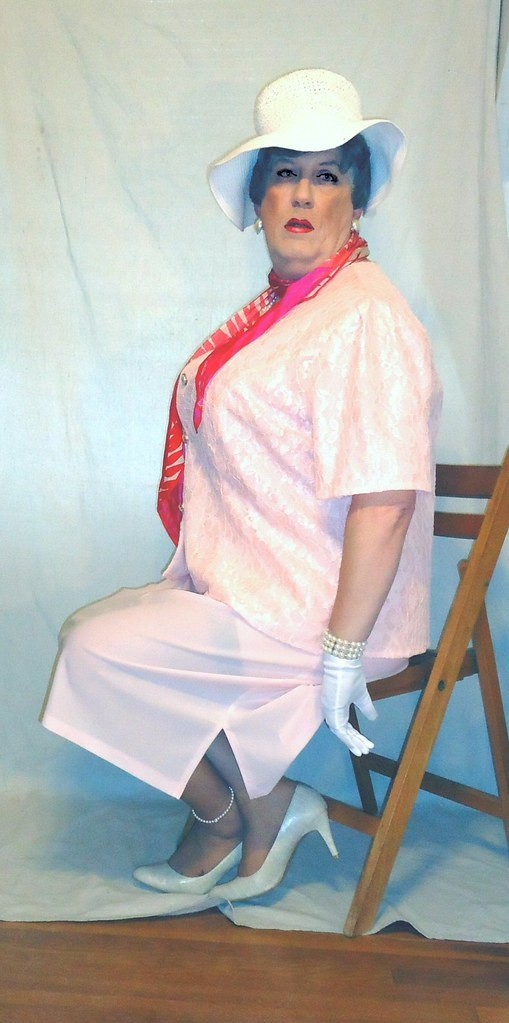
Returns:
[321,651,378,757]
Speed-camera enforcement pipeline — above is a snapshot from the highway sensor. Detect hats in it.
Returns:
[208,70,408,232]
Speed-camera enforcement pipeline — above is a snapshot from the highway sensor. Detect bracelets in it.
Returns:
[320,633,367,660]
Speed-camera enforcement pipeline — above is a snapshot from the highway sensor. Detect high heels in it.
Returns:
[134,840,243,894]
[210,781,339,901]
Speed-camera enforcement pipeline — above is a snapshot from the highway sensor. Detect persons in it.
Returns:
[40,68,442,899]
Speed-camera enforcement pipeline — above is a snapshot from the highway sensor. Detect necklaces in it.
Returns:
[262,296,276,312]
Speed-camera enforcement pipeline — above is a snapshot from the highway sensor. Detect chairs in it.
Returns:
[176,449,509,938]
